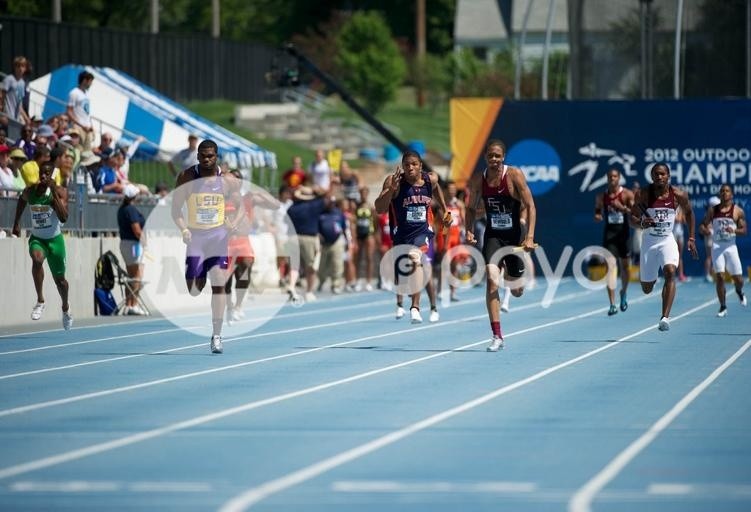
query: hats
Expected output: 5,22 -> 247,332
79,151 -> 101,167
295,186 -> 316,201
35,125 -> 55,137
0,145 -> 29,161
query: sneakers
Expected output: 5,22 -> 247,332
739,296 -> 747,307
226,278 -> 459,324
717,308 -> 728,318
608,305 -> 618,316
210,335 -> 224,354
658,317 -> 671,332
62,301 -> 73,331
487,335 -> 505,352
122,304 -> 150,316
500,301 -> 509,313
31,301 -> 46,320
619,289 -> 628,312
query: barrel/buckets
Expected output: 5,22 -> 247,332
359,149 -> 377,161
383,144 -> 401,162
408,142 -> 424,158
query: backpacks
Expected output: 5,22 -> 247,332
95,249 -> 119,292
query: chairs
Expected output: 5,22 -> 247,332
106,256 -> 152,319
